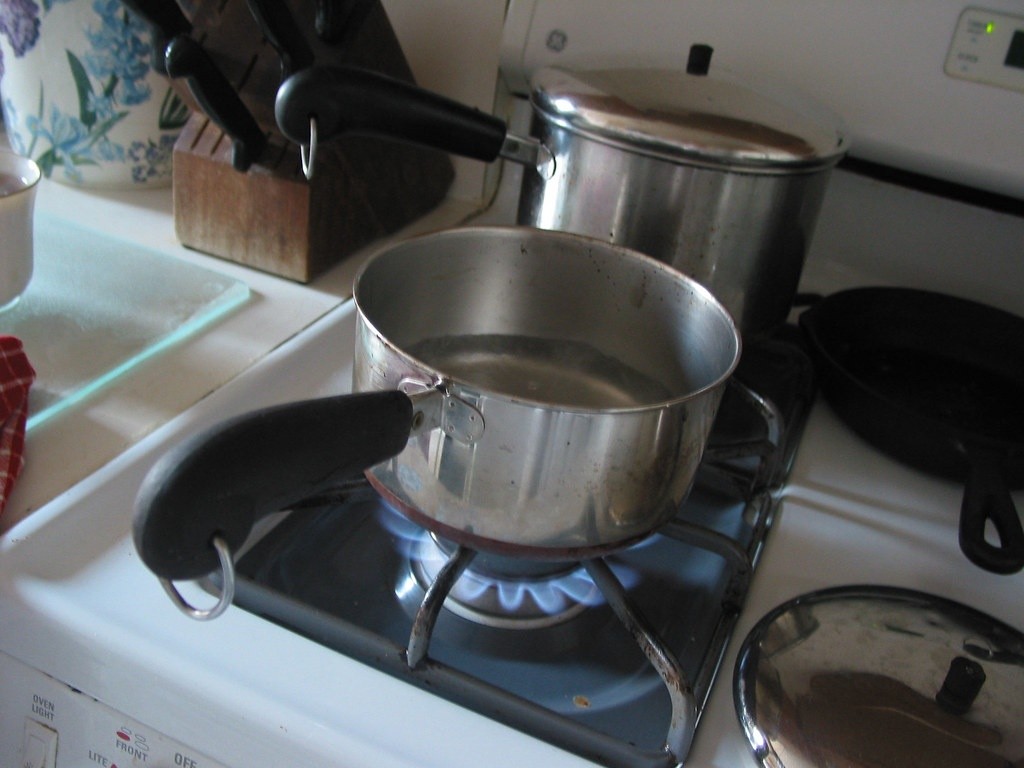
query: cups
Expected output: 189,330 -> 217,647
0,151 -> 42,306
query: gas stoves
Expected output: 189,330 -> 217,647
1,0 -> 1024,768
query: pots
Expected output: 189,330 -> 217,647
801,285 -> 1024,579
132,226 -> 743,622
271,42 -> 849,319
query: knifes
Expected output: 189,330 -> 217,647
121,0 -> 354,174
